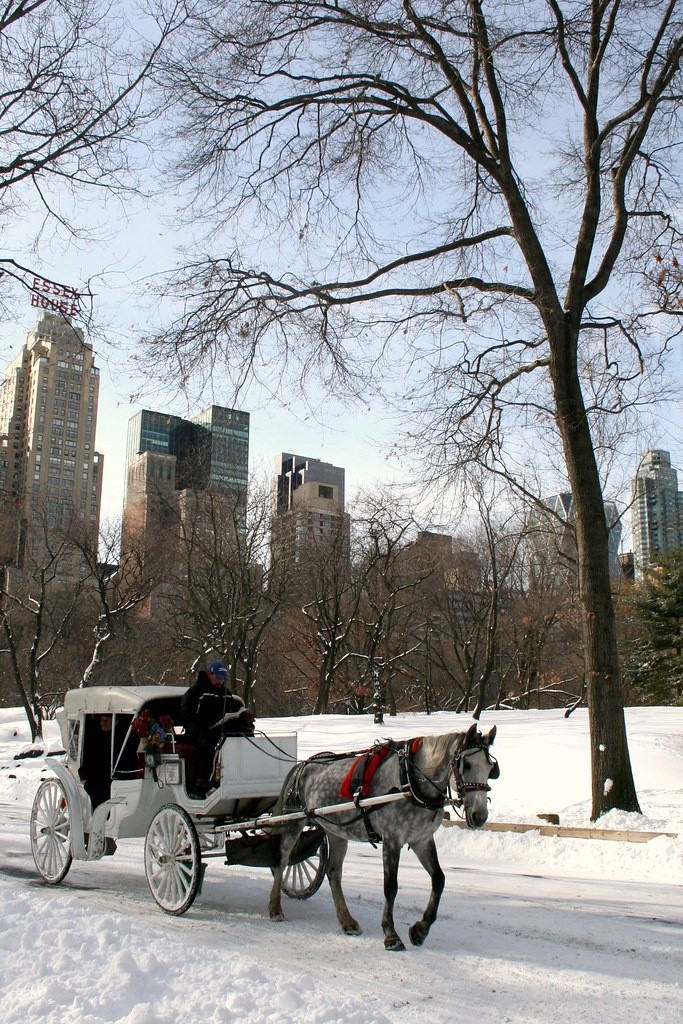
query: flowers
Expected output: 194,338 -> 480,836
131,709 -> 176,783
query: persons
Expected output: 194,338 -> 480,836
172,662 -> 253,798
82,713 -> 132,809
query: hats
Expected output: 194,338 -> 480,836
209,661 -> 228,679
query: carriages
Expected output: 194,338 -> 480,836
31,686 -> 499,952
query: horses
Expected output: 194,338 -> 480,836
269,723 -> 496,952
